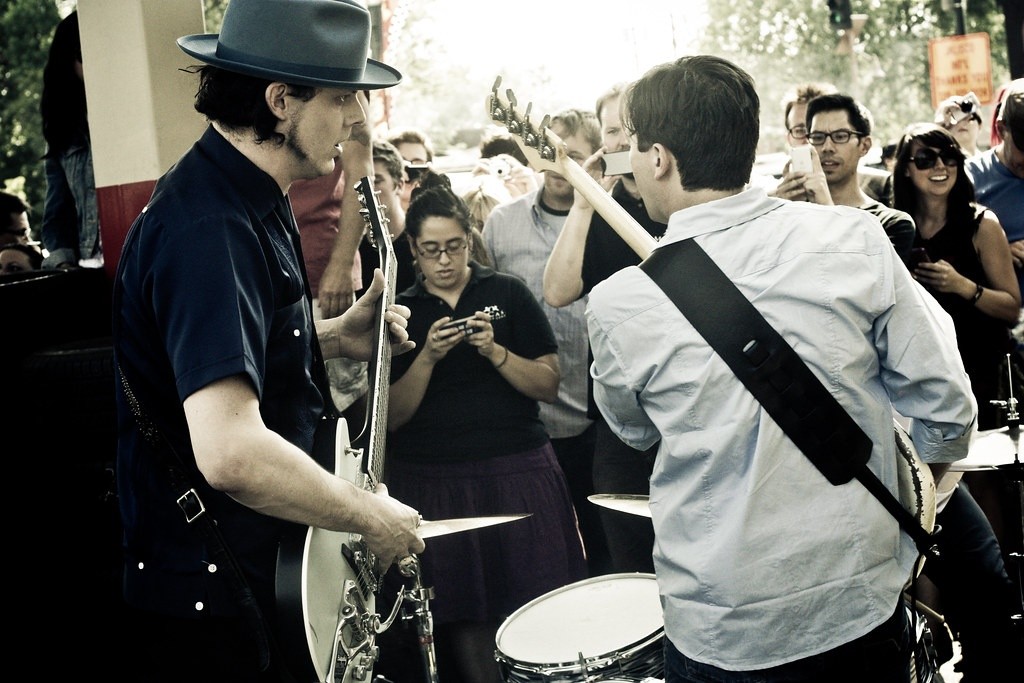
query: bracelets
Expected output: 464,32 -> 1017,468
495,347 -> 508,368
969,284 -> 983,304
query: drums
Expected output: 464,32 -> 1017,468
492,570 -> 665,683
903,598 -> 940,683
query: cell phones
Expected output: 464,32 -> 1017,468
439,315 -> 482,341
911,247 -> 931,270
601,150 -> 633,176
789,145 -> 813,174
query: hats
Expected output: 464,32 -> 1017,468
1000,78 -> 1024,152
880,144 -> 898,157
176,0 -> 402,89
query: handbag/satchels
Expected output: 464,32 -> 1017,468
997,352 -> 1024,428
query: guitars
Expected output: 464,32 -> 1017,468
483,71 -> 938,591
273,172 -> 406,683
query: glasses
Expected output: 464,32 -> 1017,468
789,127 -> 808,139
908,146 -> 960,169
806,129 -> 863,145
402,157 -> 424,165
414,235 -> 470,259
10,228 -> 30,240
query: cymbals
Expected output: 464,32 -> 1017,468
412,511 -> 537,540
586,491 -> 652,518
935,422 -> 1024,466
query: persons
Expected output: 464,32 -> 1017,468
111,1 -> 424,683
585,55 -> 978,683
0,9 -> 1024,683
